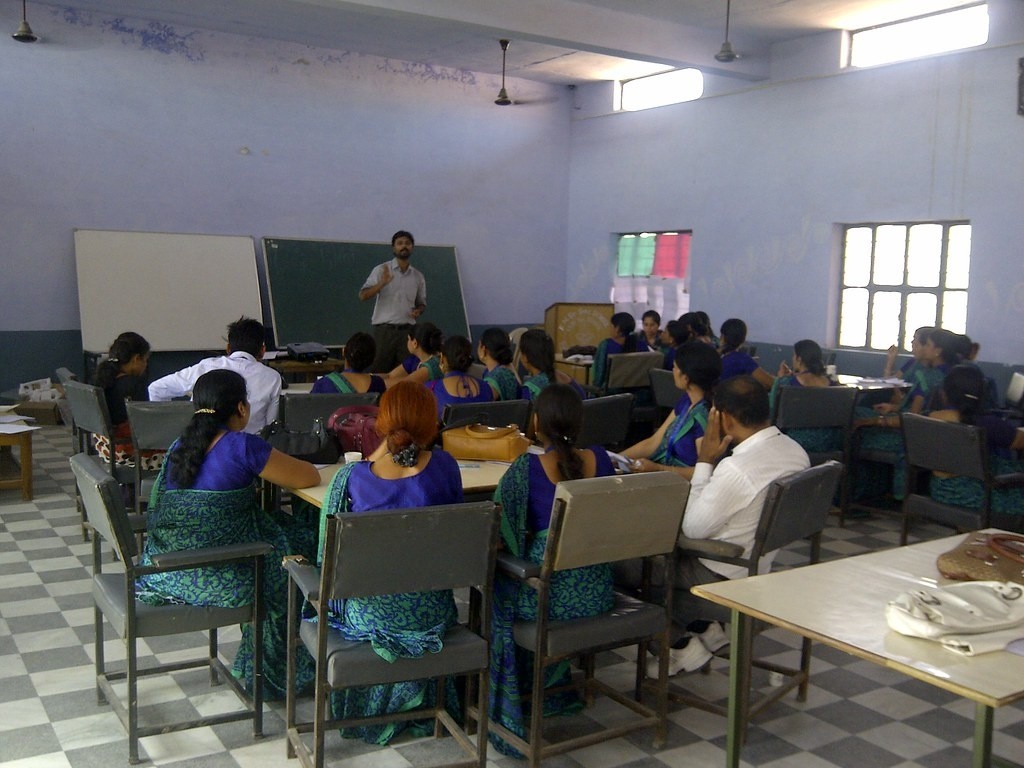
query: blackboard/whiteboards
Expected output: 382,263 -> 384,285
261,236 -> 472,350
73,226 -> 265,353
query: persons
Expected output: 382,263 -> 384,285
136,369 -> 321,700
148,319 -> 283,435
587,311 -> 775,421
928,365 -> 1024,513
770,328 -> 998,518
645,375 -> 811,679
613,341 -> 729,479
310,322 -> 586,432
302,381 -> 466,745
359,230 -> 428,374
473,384 -> 616,758
93,332 -> 166,512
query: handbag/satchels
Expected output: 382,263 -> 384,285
262,417 -> 339,464
937,530 -> 1024,584
885,581 -> 1024,656
326,405 -> 384,459
441,423 -> 530,463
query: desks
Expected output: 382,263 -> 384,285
690,525 -> 1023,768
838,373 -> 913,393
260,442 -> 546,511
0,405 -> 34,501
269,357 -> 342,375
279,382 -> 316,396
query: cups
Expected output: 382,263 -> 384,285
345,452 -> 363,465
826,364 -> 838,376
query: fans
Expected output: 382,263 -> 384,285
454,39 -> 562,106
658,0 -> 802,67
0,0 -> 103,51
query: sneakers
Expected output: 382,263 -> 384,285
646,637 -> 714,680
693,622 -> 731,655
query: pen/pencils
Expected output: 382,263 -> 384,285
458,464 -> 480,468
624,455 -> 634,466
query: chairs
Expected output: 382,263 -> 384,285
54,327 -> 1023,768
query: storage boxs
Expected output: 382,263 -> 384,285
1,387 -> 61,428
